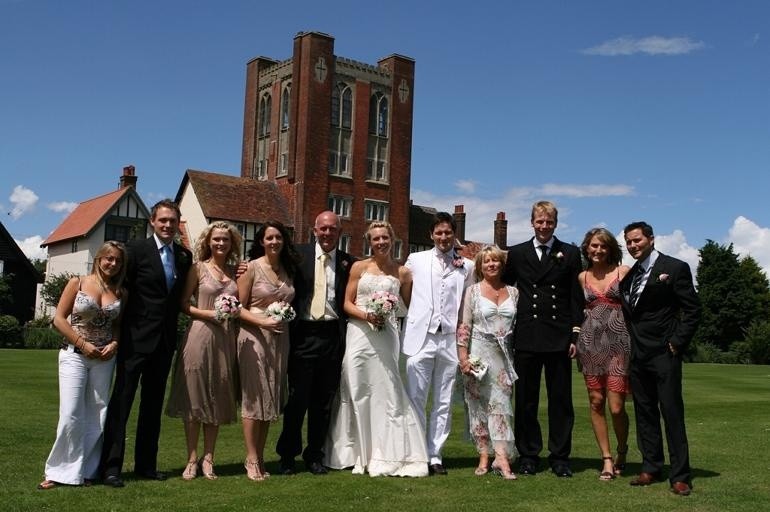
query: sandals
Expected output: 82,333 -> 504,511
182,459 -> 200,480
599,454 -> 629,482
202,459 -> 219,481
244,458 -> 270,481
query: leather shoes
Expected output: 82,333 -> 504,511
98,472 -> 126,487
304,461 -> 328,475
671,480 -> 691,495
135,468 -> 169,482
281,457 -> 296,475
628,471 -> 665,487
548,458 -> 573,478
519,455 -> 542,475
429,457 -> 449,477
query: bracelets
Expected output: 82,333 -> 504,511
364,311 -> 368,323
74,335 -> 81,346
366,313 -> 370,321
371,311 -> 373,320
457,358 -> 471,364
80,340 -> 86,352
107,341 -> 118,354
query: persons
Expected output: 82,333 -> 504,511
161,221 -> 247,481
498,200 -> 586,478
98,200 -> 194,486
575,225 -> 635,483
453,242 -> 524,481
400,209 -> 481,476
35,239 -> 130,490
234,208 -> 360,477
319,218 -> 431,478
234,217 -> 297,484
615,219 -> 706,497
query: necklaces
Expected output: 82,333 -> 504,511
209,260 -> 229,282
488,285 -> 505,300
267,267 -> 282,286
98,281 -> 110,290
372,258 -> 392,275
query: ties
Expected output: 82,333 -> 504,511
539,245 -> 551,271
308,253 -> 331,321
628,264 -> 645,306
440,255 -> 449,272
162,246 -> 174,288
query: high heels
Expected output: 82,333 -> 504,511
474,461 -> 517,481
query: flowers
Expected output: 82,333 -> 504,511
213,290 -> 242,324
263,298 -> 298,323
364,289 -> 402,334
